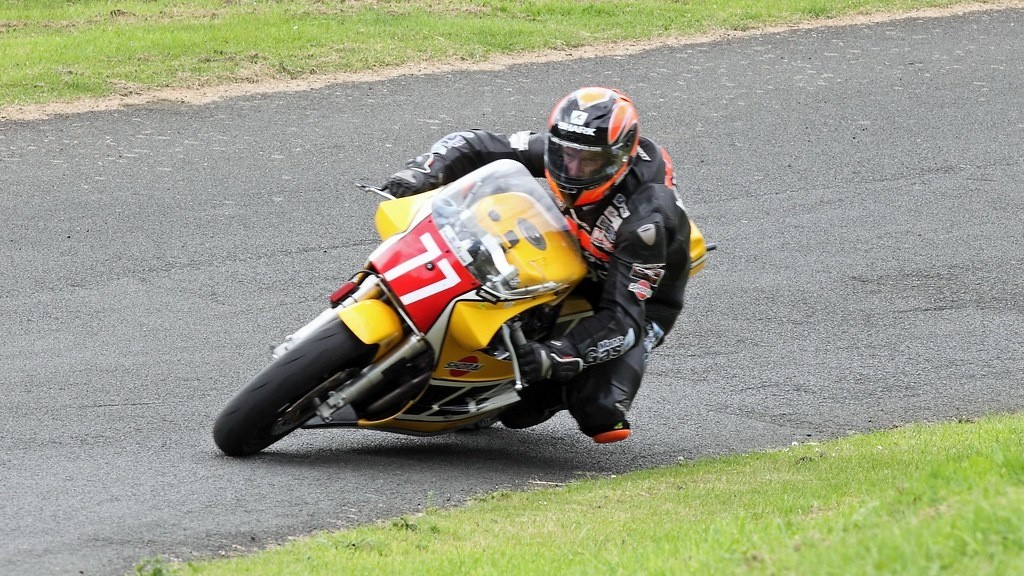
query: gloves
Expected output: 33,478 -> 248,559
517,336 -> 582,382
385,152 -> 434,195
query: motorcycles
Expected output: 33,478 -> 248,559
213,156 -> 717,457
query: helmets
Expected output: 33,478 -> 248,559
544,86 -> 638,207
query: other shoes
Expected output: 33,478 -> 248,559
502,383 -> 566,429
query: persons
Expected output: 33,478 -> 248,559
378,86 -> 692,445
